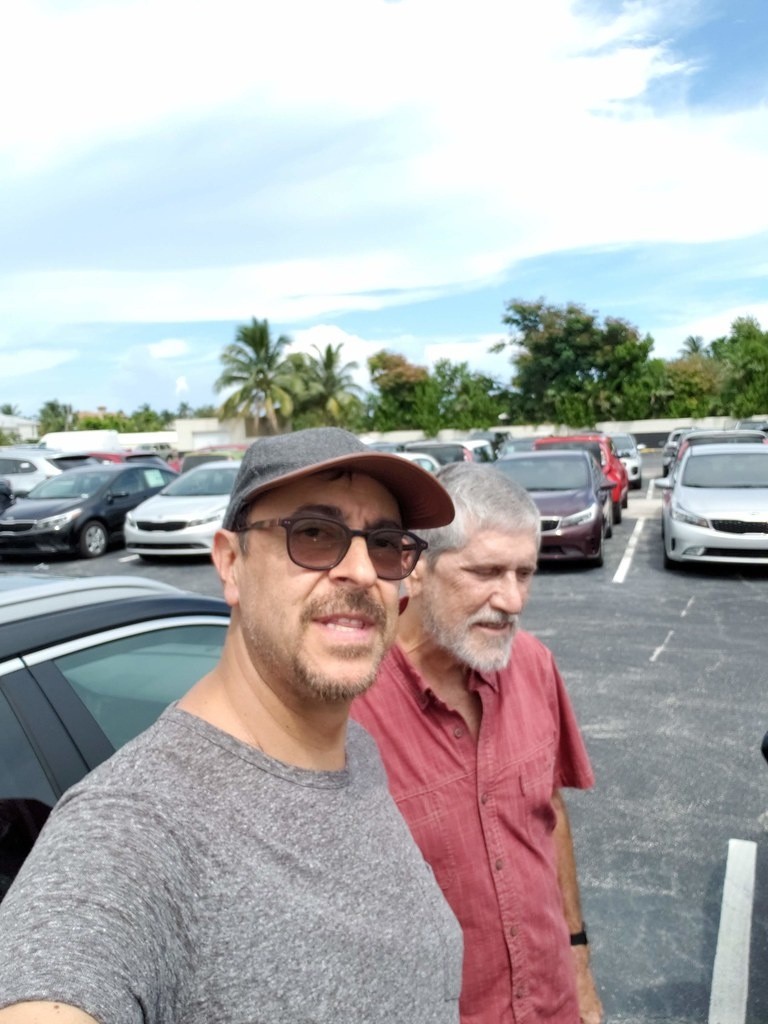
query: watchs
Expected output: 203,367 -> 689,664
569,921 -> 589,946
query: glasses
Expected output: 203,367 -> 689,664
236,513 -> 430,578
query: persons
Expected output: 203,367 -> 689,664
0,431 -> 467,1024
352,460 -> 607,1024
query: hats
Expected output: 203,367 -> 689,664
222,425 -> 457,532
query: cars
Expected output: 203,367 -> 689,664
2,398 -> 766,916
